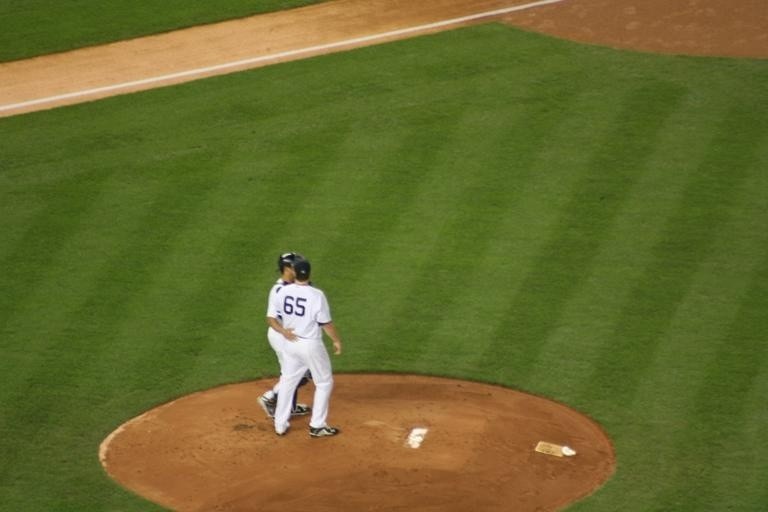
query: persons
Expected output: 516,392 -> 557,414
258,252 -> 341,437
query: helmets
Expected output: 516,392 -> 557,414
279,253 -> 310,278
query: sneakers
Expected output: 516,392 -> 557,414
308,427 -> 339,437
257,390 -> 312,435
